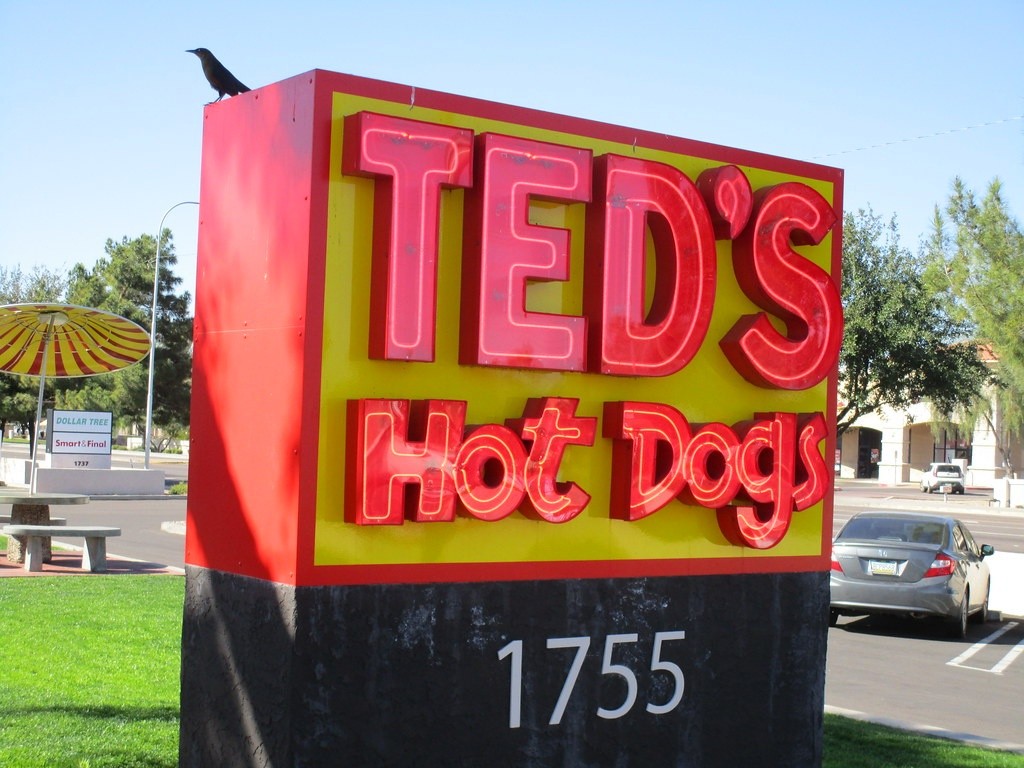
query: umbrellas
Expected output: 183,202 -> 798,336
0,301 -> 153,494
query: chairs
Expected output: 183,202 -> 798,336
913,527 -> 939,544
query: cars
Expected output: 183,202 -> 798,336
920,463 -> 966,495
830,511 -> 995,638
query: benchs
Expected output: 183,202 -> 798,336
3,525 -> 121,572
0,514 -> 67,526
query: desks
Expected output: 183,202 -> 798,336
0,491 -> 89,564
